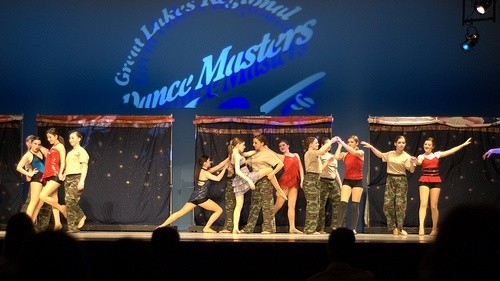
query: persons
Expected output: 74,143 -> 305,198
154,154 -> 230,233
218,131 -> 364,235
16,128 -> 90,233
360,134 -> 472,237
2,211 -> 372,281
482,147 -> 500,160
